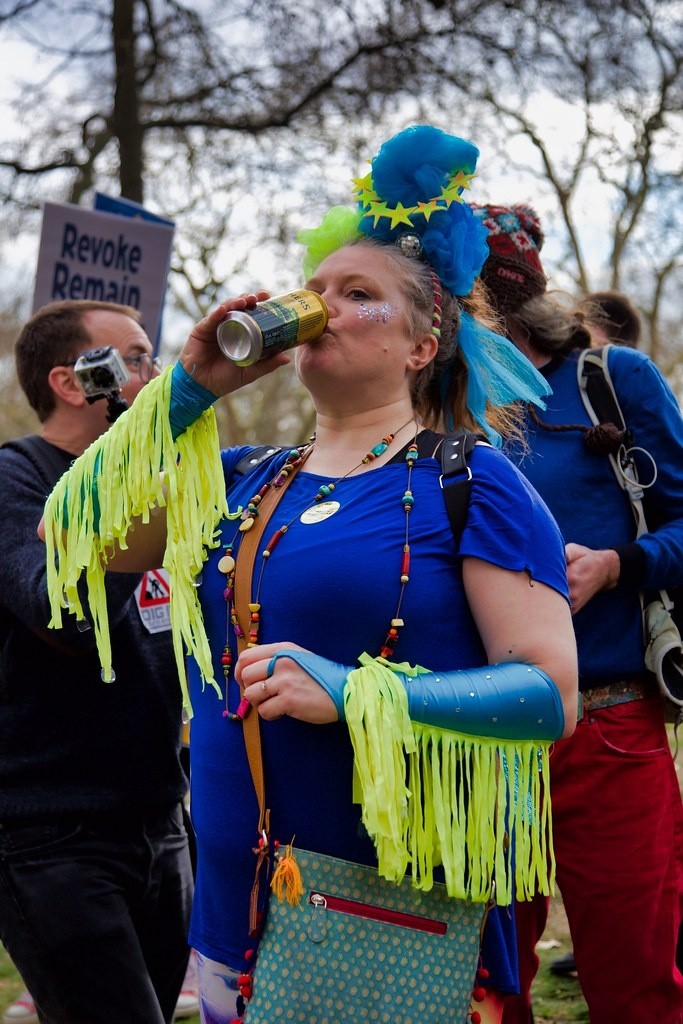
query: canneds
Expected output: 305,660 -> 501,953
217,289 -> 330,366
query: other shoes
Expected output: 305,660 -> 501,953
3,989 -> 37,1023
550,952 -> 578,977
175,985 -> 200,1019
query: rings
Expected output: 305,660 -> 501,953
262,681 -> 271,699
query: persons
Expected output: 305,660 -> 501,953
39,234 -> 579,1024
483,204 -> 682,1024
1,299 -> 193,1023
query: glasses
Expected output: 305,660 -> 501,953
62,352 -> 162,384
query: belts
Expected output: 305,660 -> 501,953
577,682 -> 647,721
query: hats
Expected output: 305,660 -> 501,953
468,201 -> 548,309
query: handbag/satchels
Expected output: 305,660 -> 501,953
243,844 -> 495,1024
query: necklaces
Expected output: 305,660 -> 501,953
218,423 -> 418,668
223,419 -> 415,720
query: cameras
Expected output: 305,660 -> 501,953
72,346 -> 131,426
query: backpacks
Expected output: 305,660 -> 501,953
577,343 -> 683,722
